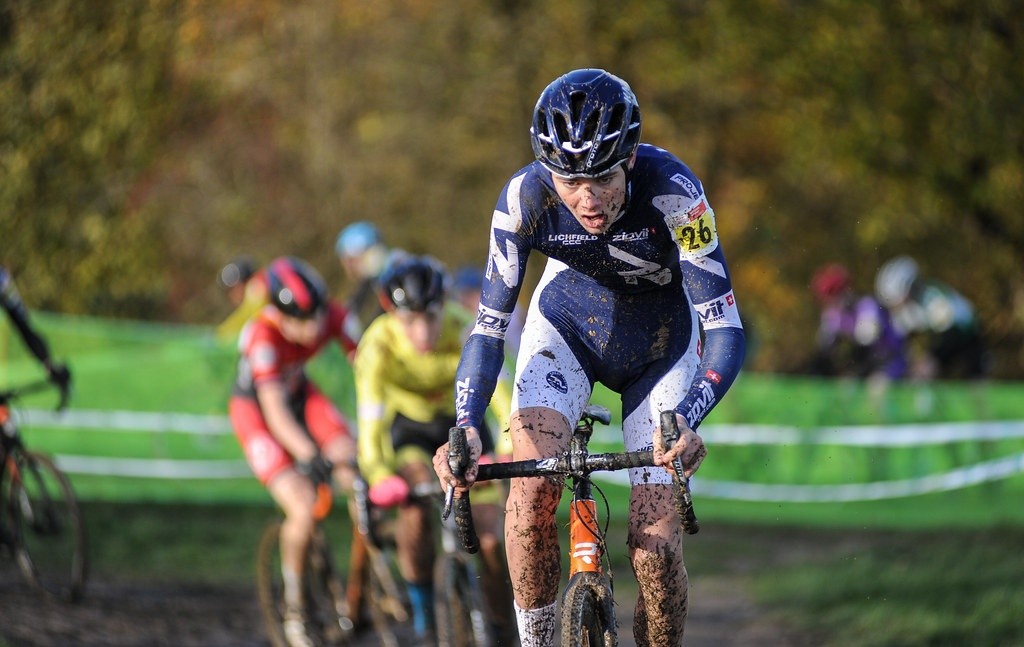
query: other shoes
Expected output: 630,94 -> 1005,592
284,606 -> 321,647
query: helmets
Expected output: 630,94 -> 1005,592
262,254 -> 327,319
530,67 -> 641,177
876,256 -> 918,305
381,254 -> 445,314
814,264 -> 848,297
456,266 -> 480,290
338,221 -> 378,255
221,261 -> 251,291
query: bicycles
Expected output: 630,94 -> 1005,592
253,455 -> 440,647
440,405 -> 699,647
352,475 -> 512,647
0,367 -> 93,606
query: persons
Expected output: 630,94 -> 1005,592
432,68 -> 746,647
228,220 -> 526,647
0,265 -> 68,486
812,255 -> 994,419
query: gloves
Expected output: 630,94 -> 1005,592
370,475 -> 412,508
